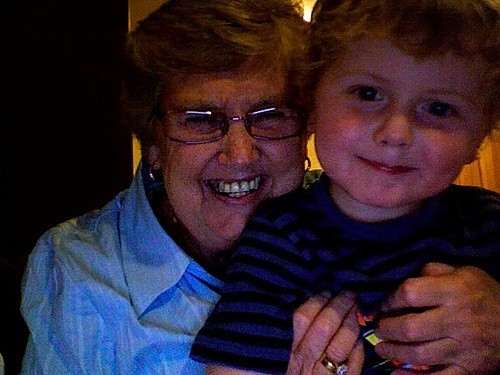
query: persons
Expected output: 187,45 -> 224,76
190,0 -> 500,375
19,0 -> 500,375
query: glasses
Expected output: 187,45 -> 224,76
148,102 -> 307,146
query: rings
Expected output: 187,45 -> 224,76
320,353 -> 341,374
